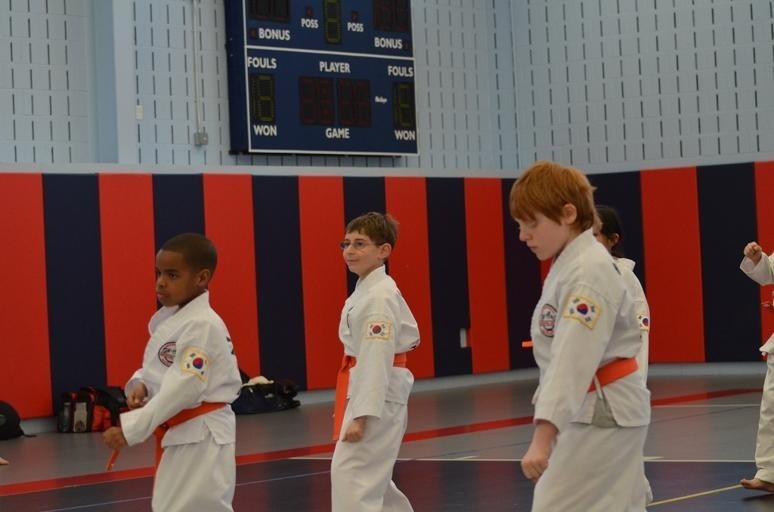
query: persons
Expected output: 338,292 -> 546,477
329,210 -> 421,512
101,231 -> 244,511
591,202 -> 654,504
738,241 -> 774,494
508,160 -> 647,512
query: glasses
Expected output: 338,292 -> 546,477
339,241 -> 374,251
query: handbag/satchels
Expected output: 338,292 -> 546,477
233,370 -> 301,414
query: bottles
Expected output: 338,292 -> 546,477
459,329 -> 470,375
62,401 -> 72,432
